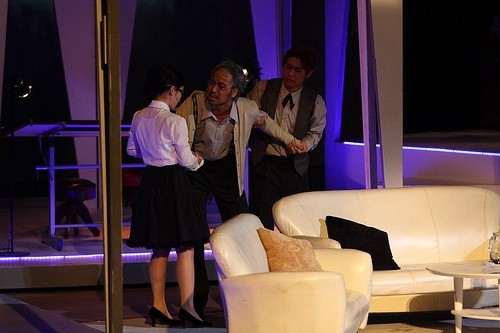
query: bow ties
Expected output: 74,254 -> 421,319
282,93 -> 295,110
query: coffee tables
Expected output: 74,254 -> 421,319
424,258 -> 500,333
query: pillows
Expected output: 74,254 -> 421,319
258,227 -> 324,273
325,214 -> 401,270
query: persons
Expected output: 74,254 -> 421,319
248,45 -> 326,232
127,71 -> 212,329
175,61 -> 306,319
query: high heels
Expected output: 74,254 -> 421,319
150,306 -> 182,328
178,307 -> 208,329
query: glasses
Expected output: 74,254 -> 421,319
207,79 -> 236,91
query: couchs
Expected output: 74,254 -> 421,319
208,213 -> 372,333
271,186 -> 500,313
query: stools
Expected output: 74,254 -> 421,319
48,176 -> 101,240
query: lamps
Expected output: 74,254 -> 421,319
0,73 -> 32,257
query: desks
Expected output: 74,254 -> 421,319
5,119 -> 132,252
